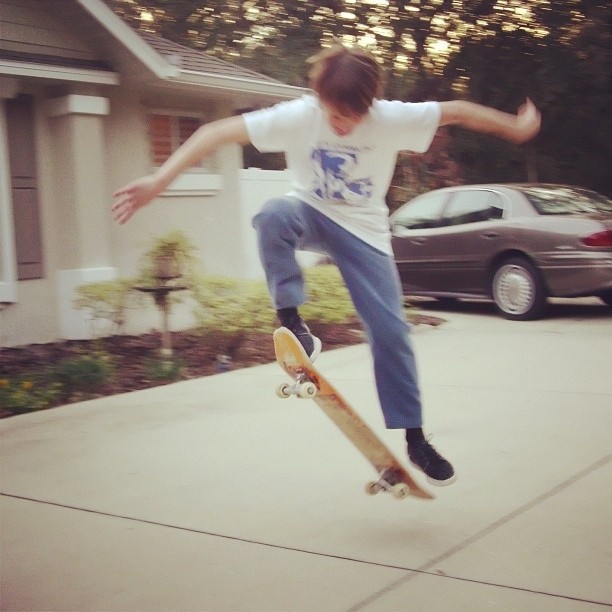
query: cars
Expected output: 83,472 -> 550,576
313,181 -> 612,322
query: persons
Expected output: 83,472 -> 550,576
111,47 -> 541,486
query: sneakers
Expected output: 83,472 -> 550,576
286,316 -> 323,363
407,432 -> 457,485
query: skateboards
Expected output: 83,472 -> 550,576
273,327 -> 436,500
